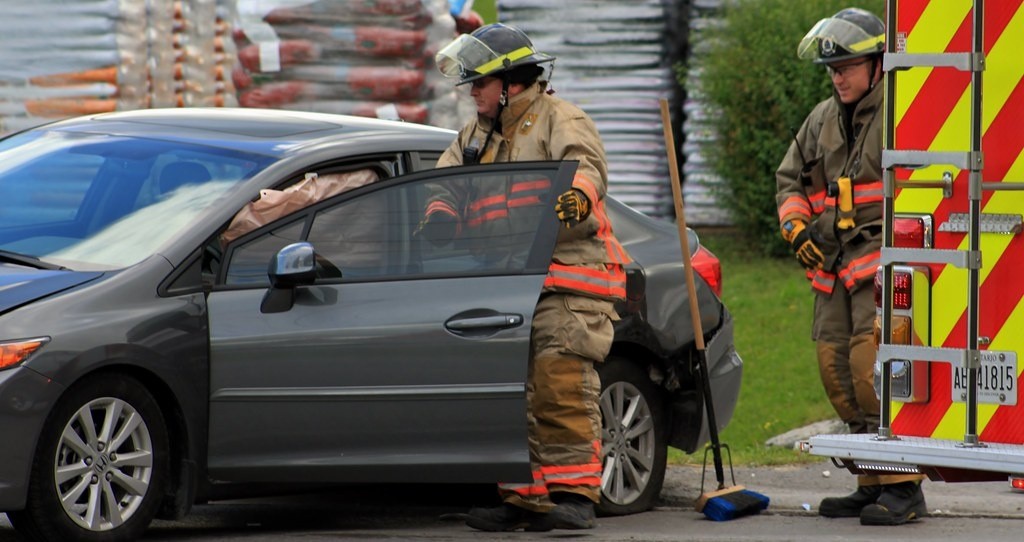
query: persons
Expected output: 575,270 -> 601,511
412,22 -> 635,533
775,8 -> 927,526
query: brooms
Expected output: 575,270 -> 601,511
651,95 -> 769,522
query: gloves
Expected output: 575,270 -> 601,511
782,219 -> 825,274
413,211 -> 459,248
555,188 -> 590,229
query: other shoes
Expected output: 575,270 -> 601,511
818,484 -> 882,517
548,493 -> 598,529
860,480 -> 927,525
465,502 -> 555,532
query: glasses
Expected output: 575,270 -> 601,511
825,59 -> 871,78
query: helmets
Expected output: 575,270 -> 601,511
797,7 -> 885,63
435,23 -> 557,86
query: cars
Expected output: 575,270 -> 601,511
0,105 -> 745,541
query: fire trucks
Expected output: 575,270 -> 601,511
759,1 -> 1024,496
